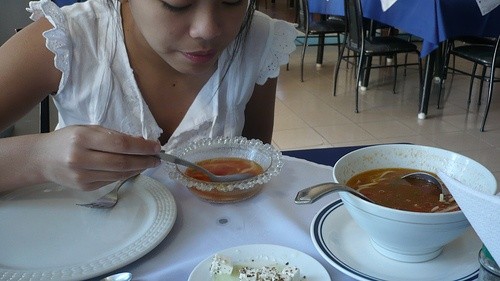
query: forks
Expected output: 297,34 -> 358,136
73,149 -> 166,210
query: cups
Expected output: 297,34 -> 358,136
477,242 -> 500,281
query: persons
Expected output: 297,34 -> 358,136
0,0 -> 301,193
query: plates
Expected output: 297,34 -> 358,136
187,244 -> 331,281
310,197 -> 484,281
0,172 -> 178,281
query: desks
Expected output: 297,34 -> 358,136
308,0 -> 500,119
91,142 -> 412,281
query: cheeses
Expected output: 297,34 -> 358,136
210,254 -> 300,281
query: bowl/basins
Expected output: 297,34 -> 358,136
164,135 -> 284,204
332,144 -> 498,264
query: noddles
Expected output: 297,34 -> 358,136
346,168 -> 462,212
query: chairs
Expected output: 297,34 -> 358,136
286,0 -> 500,132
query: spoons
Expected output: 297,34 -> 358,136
100,272 -> 133,281
154,153 -> 255,182
